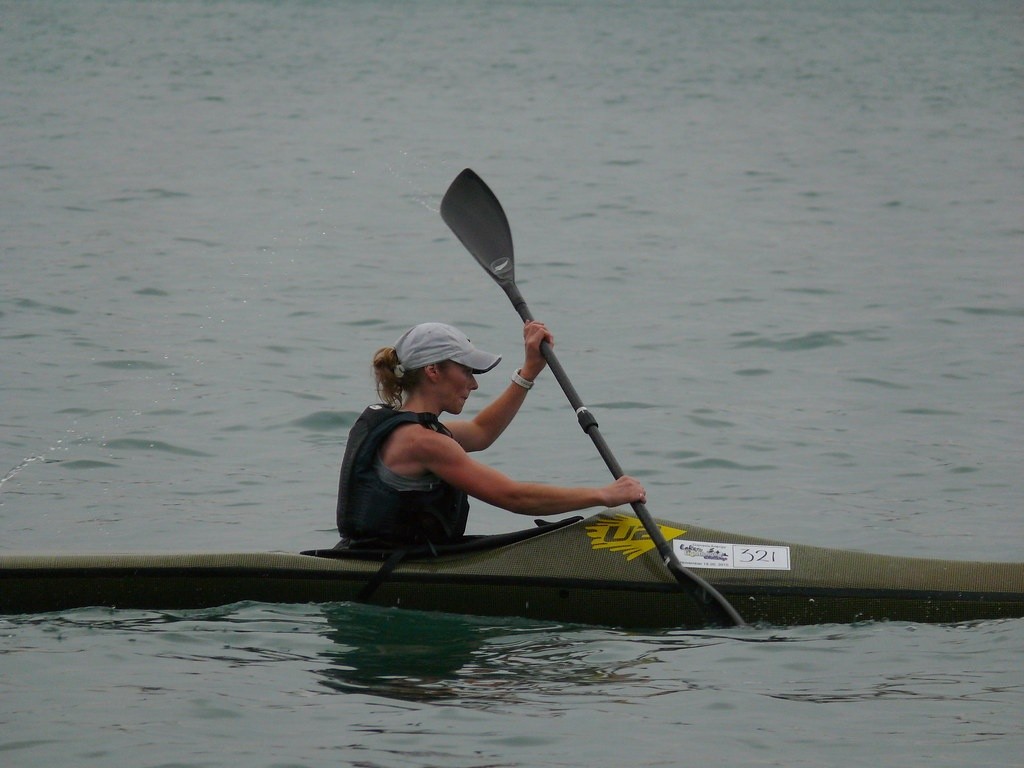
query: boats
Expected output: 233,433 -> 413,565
0,502 -> 1024,626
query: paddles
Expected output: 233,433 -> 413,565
438,165 -> 749,628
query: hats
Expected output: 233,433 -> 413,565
393,322 -> 503,374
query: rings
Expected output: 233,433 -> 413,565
639,492 -> 642,496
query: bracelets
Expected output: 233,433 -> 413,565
512,368 -> 534,390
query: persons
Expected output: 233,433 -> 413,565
335,320 -> 647,551
